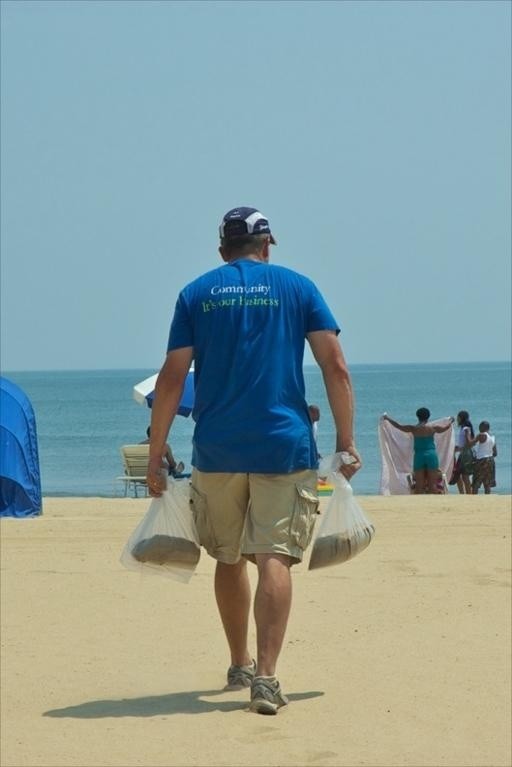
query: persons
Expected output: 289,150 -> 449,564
145,204 -> 364,716
139,425 -> 185,473
465,420 -> 498,494
454,410 -> 477,494
308,404 -> 324,460
383,407 -> 455,494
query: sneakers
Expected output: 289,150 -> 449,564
227,658 -> 290,716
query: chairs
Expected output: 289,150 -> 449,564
117,443 -> 151,497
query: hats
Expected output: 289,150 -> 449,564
219,207 -> 278,246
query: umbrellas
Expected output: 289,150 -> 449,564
131,368 -> 195,420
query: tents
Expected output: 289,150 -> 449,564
2,376 -> 45,525
